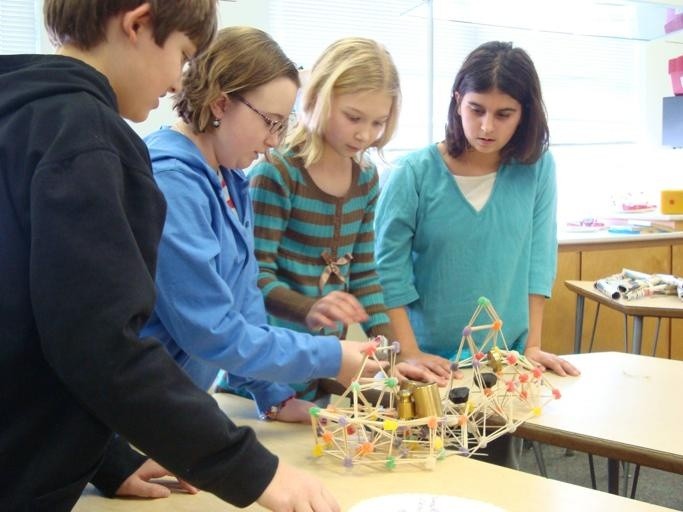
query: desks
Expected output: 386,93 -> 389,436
71,392 -> 669,508
321,351 -> 683,499
565,279 -> 683,460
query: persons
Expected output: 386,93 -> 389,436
373,40 -> 582,381
0,0 -> 343,512
247,37 -> 448,388
142,25 -> 389,424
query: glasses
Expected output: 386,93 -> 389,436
229,91 -> 288,137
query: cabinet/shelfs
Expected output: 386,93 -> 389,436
671,246 -> 683,360
539,253 -> 580,354
580,243 -> 671,357
